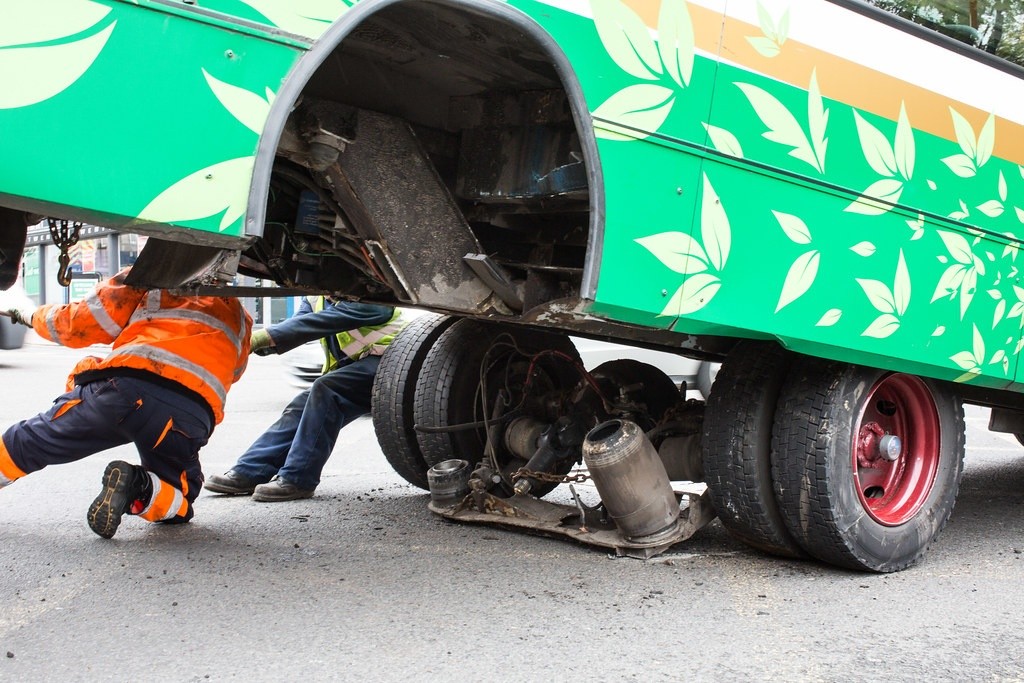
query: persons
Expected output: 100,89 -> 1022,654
0,265 -> 254,539
204,295 -> 412,502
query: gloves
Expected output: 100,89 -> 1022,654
9,306 -> 34,328
248,329 -> 269,354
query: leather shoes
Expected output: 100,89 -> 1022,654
206,471 -> 254,497
255,478 -> 315,502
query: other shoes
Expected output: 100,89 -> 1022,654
86,460 -> 148,540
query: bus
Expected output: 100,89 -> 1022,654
0,0 -> 1024,584
